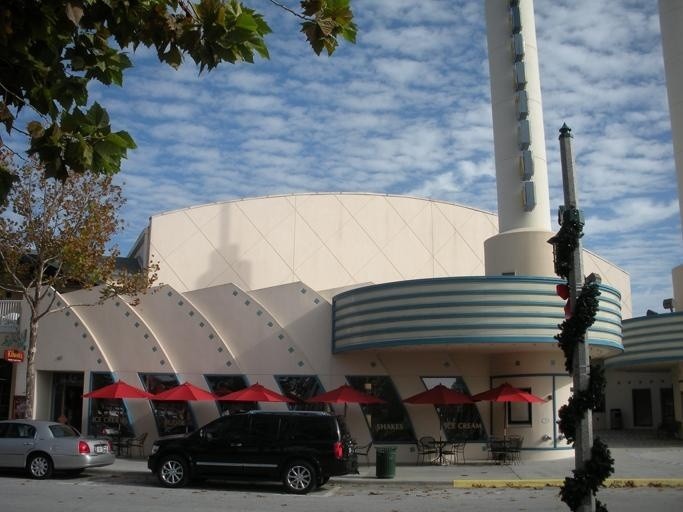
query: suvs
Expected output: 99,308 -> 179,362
146,407 -> 341,494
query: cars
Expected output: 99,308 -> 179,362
0,418 -> 116,479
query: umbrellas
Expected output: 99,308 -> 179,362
146,381 -> 221,434
302,383 -> 385,418
399,382 -> 480,441
471,381 -> 548,448
215,381 -> 298,410
81,378 -> 155,456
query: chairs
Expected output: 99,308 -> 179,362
416,432 -> 467,465
485,432 -> 524,466
353,440 -> 372,470
94,431 -> 147,461
